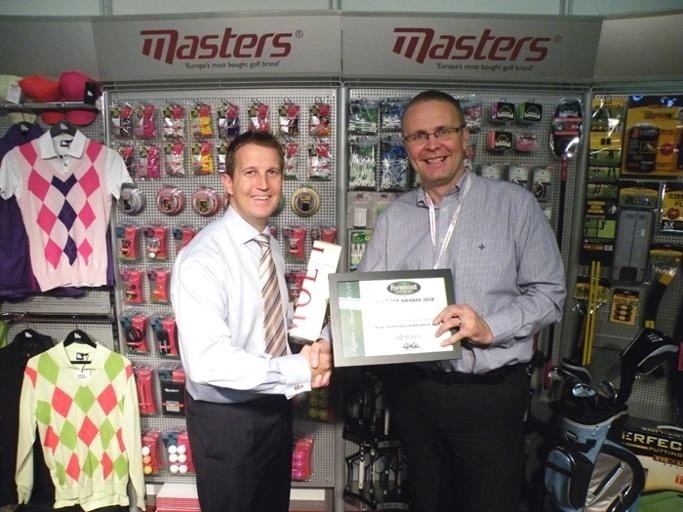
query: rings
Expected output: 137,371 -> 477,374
457,318 -> 464,326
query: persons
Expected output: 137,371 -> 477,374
170,132 -> 333,511
309,90 -> 567,510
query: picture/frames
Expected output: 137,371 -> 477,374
328,268 -> 462,369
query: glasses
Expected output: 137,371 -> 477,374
405,124 -> 466,142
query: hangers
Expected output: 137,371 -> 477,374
63,313 -> 97,364
20,309 -> 40,344
16,101 -> 34,132
50,102 -> 77,137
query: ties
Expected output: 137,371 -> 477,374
252,234 -> 287,359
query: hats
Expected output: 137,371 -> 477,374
59,72 -> 101,125
18,74 -> 64,125
0,74 -> 38,124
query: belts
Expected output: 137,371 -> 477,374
411,362 -> 527,385
